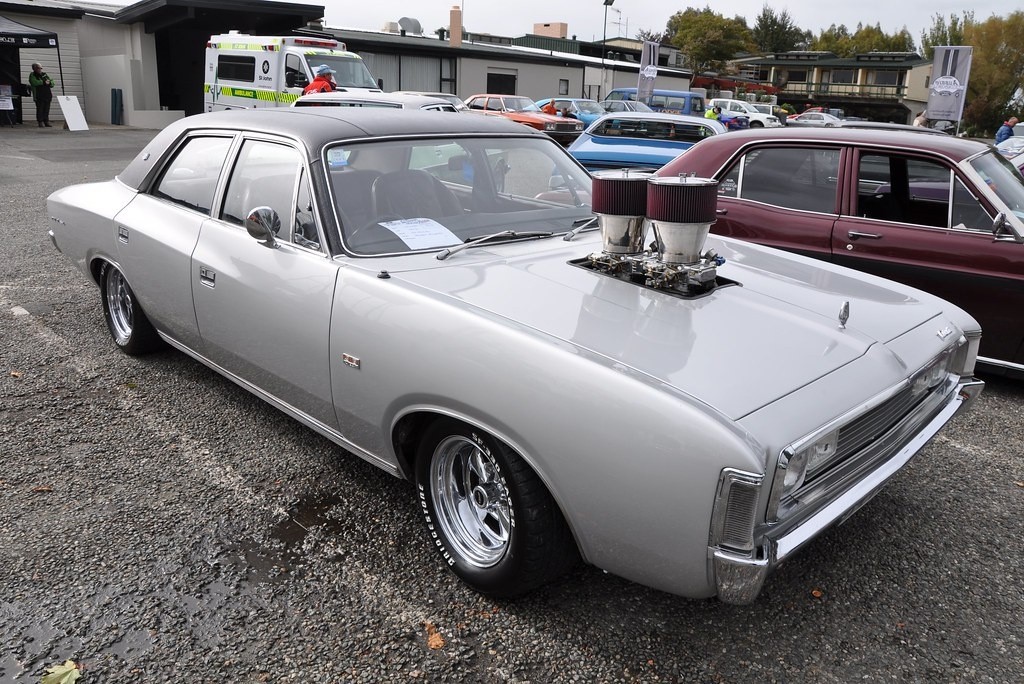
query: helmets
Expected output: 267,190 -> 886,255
32,63 -> 42,70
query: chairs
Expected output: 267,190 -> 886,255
372,167 -> 464,228
332,167 -> 386,230
242,174 -> 297,241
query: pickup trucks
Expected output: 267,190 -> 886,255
786,107 -> 822,119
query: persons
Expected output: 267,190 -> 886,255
29,63 -> 56,127
302,64 -> 340,106
912,109 -> 928,127
543,99 -> 557,115
701,106 -> 721,135
561,107 -> 577,119
995,117 -> 1018,145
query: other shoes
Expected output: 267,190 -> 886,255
38,122 -> 43,127
44,122 -> 52,127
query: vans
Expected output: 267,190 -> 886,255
604,89 -> 705,118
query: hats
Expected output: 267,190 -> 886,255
715,106 -> 721,114
317,65 -> 336,75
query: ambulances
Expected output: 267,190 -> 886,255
203,29 -> 384,114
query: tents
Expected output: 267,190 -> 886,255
0,14 -> 65,96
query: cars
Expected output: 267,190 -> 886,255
289,93 -> 460,113
654,129 -> 1024,372
42,113 -> 982,613
524,99 -> 622,136
559,111 -> 727,170
785,111 -> 842,128
598,99 -> 654,112
709,99 -> 783,128
456,93 -> 585,146
393,90 -> 498,116
